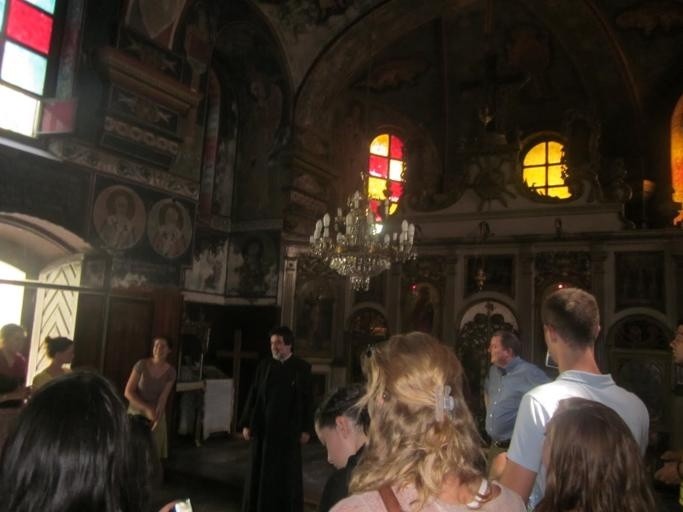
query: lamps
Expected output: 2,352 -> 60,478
308,188 -> 418,292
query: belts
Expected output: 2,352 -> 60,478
494,440 -> 509,448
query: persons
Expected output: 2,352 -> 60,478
405,287 -> 432,332
491,287 -> 651,512
314,382 -> 372,512
653,319 -> 682,512
123,336 -> 176,488
0,370 -> 191,512
150,203 -> 189,254
239,326 -> 313,512
531,396 -> 683,512
327,331 -> 530,512
99,188 -> 141,249
0,324 -> 29,414
482,330 -> 552,456
32,336 -> 76,389
